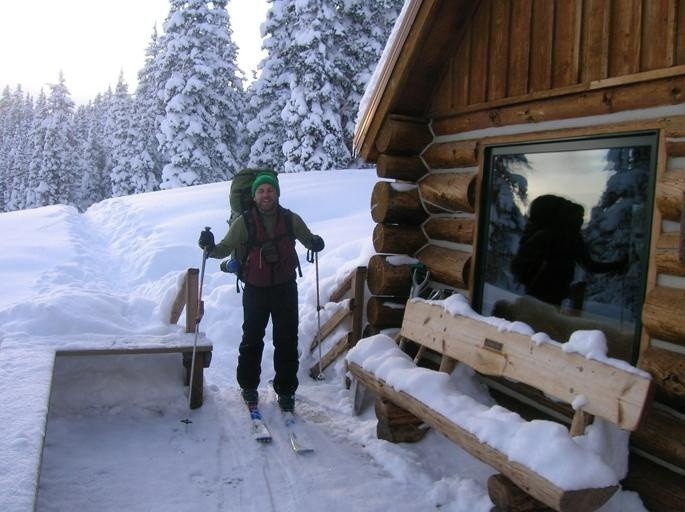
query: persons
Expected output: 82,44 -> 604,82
526,218 -> 614,306
198,170 -> 326,410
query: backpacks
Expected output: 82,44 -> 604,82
227,167 -> 294,283
512,193 -> 577,287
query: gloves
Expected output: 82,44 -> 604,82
309,235 -> 325,252
614,257 -> 633,276
198,231 -> 214,253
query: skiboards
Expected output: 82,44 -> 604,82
248,379 -> 314,454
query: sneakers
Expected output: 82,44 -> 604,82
277,392 -> 295,412
241,388 -> 259,404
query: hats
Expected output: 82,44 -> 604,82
572,203 -> 584,220
252,171 -> 281,198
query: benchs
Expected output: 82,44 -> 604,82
346,297 -> 655,512
477,298 -> 636,364
